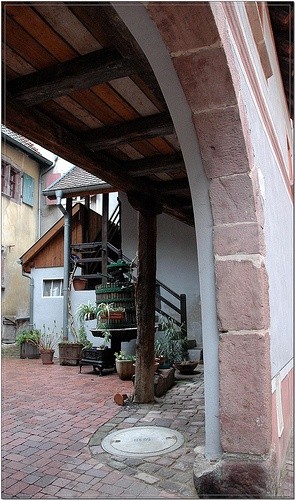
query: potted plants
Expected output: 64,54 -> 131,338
75,301 -> 97,320
58,339 -> 84,365
90,323 -> 112,341
72,277 -> 87,290
14,328 -> 41,359
40,320 -> 61,364
97,302 -> 126,321
176,359 -> 199,373
78,341 -> 117,375
161,316 -> 190,364
112,349 -> 134,380
154,350 -> 172,368
